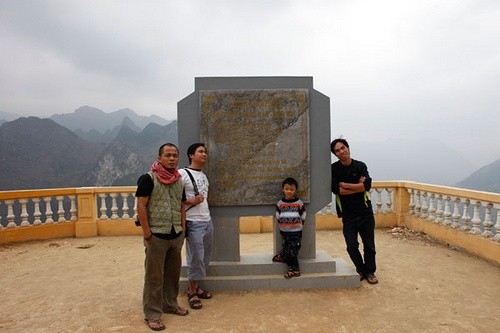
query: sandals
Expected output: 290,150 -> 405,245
272,250 -> 286,262
284,269 -> 301,278
186,286 -> 213,309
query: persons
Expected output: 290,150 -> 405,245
330,139 -> 379,285
177,142 -> 215,308
135,143 -> 189,331
272,177 -> 306,279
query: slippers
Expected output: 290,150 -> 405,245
357,271 -> 378,284
143,318 -> 166,331
163,305 -> 189,316
